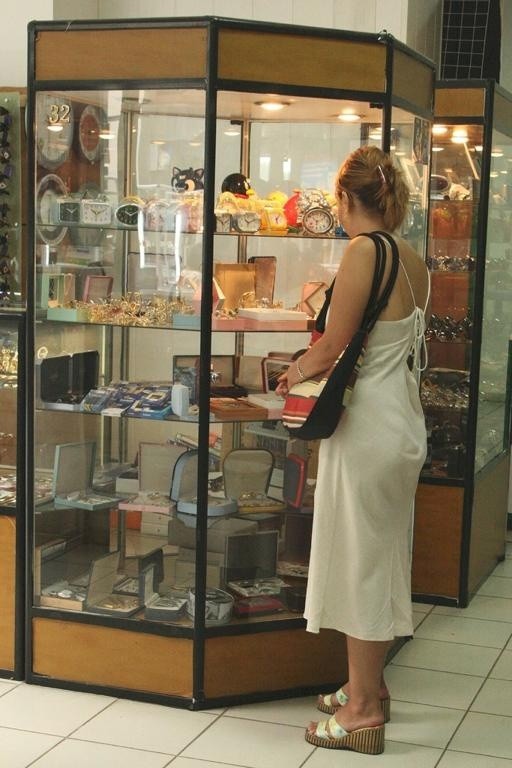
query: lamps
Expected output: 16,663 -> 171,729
45,116 -> 65,132
450,129 -> 469,145
86,118 -> 115,140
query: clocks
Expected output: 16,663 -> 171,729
55,198 -> 82,225
232,211 -> 259,233
260,205 -> 289,237
214,211 -> 232,234
297,188 -> 335,238
115,203 -> 143,228
81,199 -> 113,226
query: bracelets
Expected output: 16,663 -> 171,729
296,354 -> 311,381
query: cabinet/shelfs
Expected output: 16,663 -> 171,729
23,17 -> 437,712
403,78 -> 512,608
0,86 -> 29,682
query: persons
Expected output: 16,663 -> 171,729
271,142 -> 435,757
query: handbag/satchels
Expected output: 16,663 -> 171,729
280,325 -> 369,440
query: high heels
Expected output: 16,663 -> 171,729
305,714 -> 385,756
316,684 -> 392,724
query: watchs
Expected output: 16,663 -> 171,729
421,250 -> 476,472
